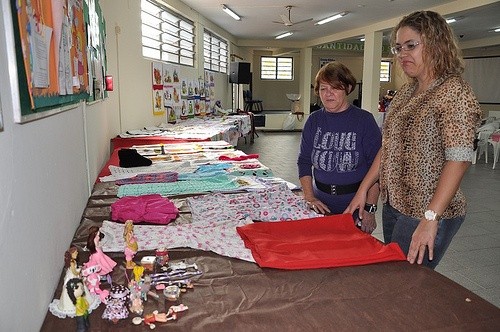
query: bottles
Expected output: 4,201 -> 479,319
156,243 -> 170,266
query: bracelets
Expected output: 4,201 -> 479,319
364,203 -> 377,212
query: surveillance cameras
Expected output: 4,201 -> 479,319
460,34 -> 464,38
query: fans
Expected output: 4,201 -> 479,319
271,6 -> 314,27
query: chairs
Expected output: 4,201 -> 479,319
469,126 -> 495,164
487,130 -> 500,169
242,90 -> 263,114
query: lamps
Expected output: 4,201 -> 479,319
274,32 -> 293,40
220,4 -> 241,21
314,11 -> 347,26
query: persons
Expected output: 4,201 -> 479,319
124,221 -> 138,267
297,62 -> 382,234
67,279 -> 89,332
102,285 -> 129,322
49,247 -> 102,318
132,308 -> 177,329
82,227 -> 117,275
343,11 -> 483,269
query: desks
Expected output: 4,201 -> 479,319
40,113 -> 500,332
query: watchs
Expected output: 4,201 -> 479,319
424,210 -> 441,221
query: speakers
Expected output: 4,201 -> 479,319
229,62 -> 251,84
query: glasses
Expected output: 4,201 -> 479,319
391,39 -> 425,55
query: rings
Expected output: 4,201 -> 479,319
312,204 -> 315,207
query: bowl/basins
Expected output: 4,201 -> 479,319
285,94 -> 301,101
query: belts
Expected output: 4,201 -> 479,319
313,178 -> 363,196
